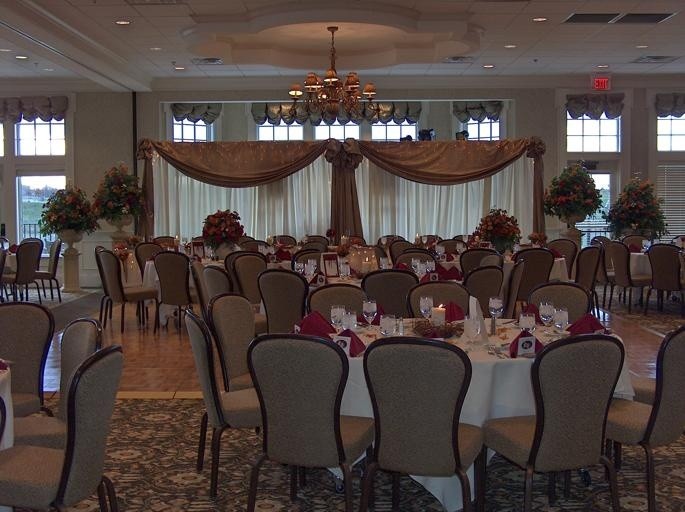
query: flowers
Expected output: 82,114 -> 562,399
469,203 -> 524,250
91,159 -> 153,224
35,184 -> 102,237
541,159 -> 602,221
600,176 -> 671,237
197,208 -> 247,246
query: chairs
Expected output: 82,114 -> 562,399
0,231 -> 685,511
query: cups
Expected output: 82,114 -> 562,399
380,237 -> 387,245
328,292 -> 569,342
293,256 -> 437,281
267,235 -> 273,245
434,239 -> 519,261
167,244 -> 278,263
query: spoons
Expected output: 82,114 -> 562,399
488,346 -> 509,359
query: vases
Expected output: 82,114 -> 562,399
56,228 -> 83,251
623,227 -> 653,236
557,214 -> 588,230
104,216 -> 135,239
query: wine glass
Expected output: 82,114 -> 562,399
422,237 -> 427,249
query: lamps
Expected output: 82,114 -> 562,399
285,22 -> 380,118
